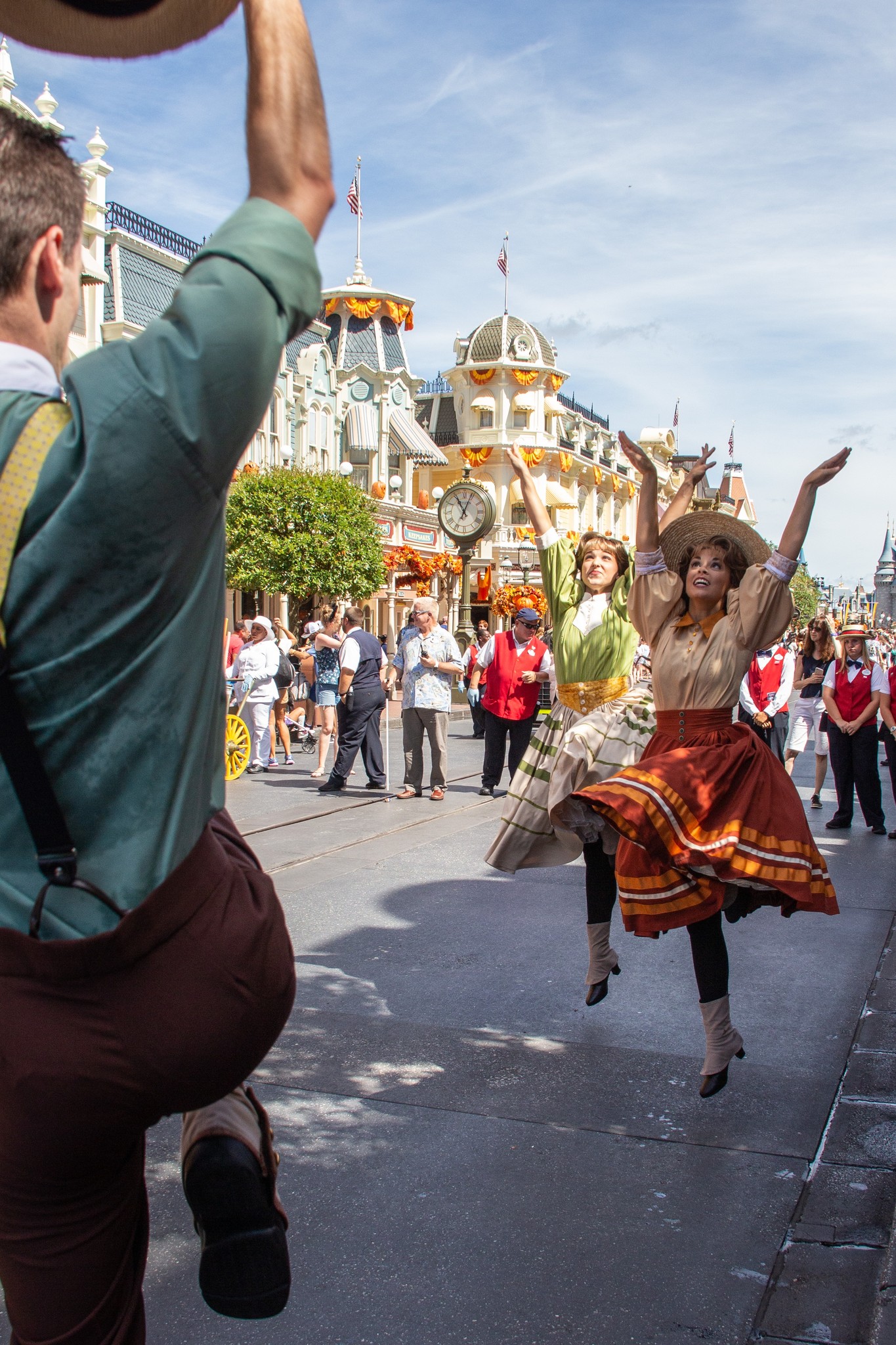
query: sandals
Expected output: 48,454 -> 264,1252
310,767 -> 355,778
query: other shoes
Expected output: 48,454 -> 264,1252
881,759 -> 889,766
887,828 -> 896,840
181,1088 -> 292,1319
479,785 -> 513,796
298,728 -> 315,739
472,733 -> 485,739
826,819 -> 887,834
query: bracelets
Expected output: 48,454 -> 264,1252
338,692 -> 347,696
292,650 -> 296,655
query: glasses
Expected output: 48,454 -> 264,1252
518,619 -> 539,629
809,626 -> 823,632
411,610 -> 428,616
478,625 -> 484,627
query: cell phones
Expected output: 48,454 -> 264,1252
815,667 -> 824,675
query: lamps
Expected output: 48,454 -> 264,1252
389,475 -> 403,496
432,486 -> 444,506
278,445 -> 294,470
339,461 -> 353,480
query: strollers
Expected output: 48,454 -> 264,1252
276,716 -> 318,754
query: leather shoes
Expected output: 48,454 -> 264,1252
396,790 -> 445,800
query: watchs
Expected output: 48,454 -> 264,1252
890,726 -> 896,734
433,661 -> 439,670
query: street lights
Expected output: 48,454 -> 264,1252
854,606 -> 871,624
810,577 -> 825,617
500,554 -> 513,631
880,610 -> 891,633
513,535 -> 539,588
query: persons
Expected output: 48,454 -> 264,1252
628,639 -> 652,691
738,634 -> 795,768
778,625 -> 896,765
784,615 -> 842,808
225,602 -> 417,792
821,625 -> 887,835
382,596 -> 465,800
879,665 -> 896,840
0,0 -> 333,1345
484,443 -> 717,1005
457,619 -> 559,739
440,616 -> 448,630
550,431 -> 853,1098
467,607 -> 551,795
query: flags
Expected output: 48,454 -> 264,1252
728,426 -> 734,457
497,241 -> 509,278
673,401 -> 679,427
347,169 -> 363,220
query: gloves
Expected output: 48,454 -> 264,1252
458,681 -> 465,693
466,688 -> 479,708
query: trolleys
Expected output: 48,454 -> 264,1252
223,678 -> 256,780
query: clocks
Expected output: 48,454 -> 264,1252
440,489 -> 484,536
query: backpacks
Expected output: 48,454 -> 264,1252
272,638 -> 311,702
783,642 -> 792,651
540,631 -> 553,653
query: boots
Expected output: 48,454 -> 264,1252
698,994 -> 746,1098
584,920 -> 621,1006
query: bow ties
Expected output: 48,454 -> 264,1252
847,660 -> 862,670
757,650 -> 772,658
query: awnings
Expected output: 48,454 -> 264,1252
388,409 -> 449,466
345,405 -> 378,453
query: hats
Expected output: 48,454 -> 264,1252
658,509 -> 773,577
515,607 -> 543,621
244,616 -> 275,640
0,0 -> 246,60
301,621 -> 324,638
835,624 -> 872,642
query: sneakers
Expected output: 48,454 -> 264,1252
319,781 -> 386,792
268,755 -> 294,766
810,795 -> 823,808
236,764 -> 269,774
